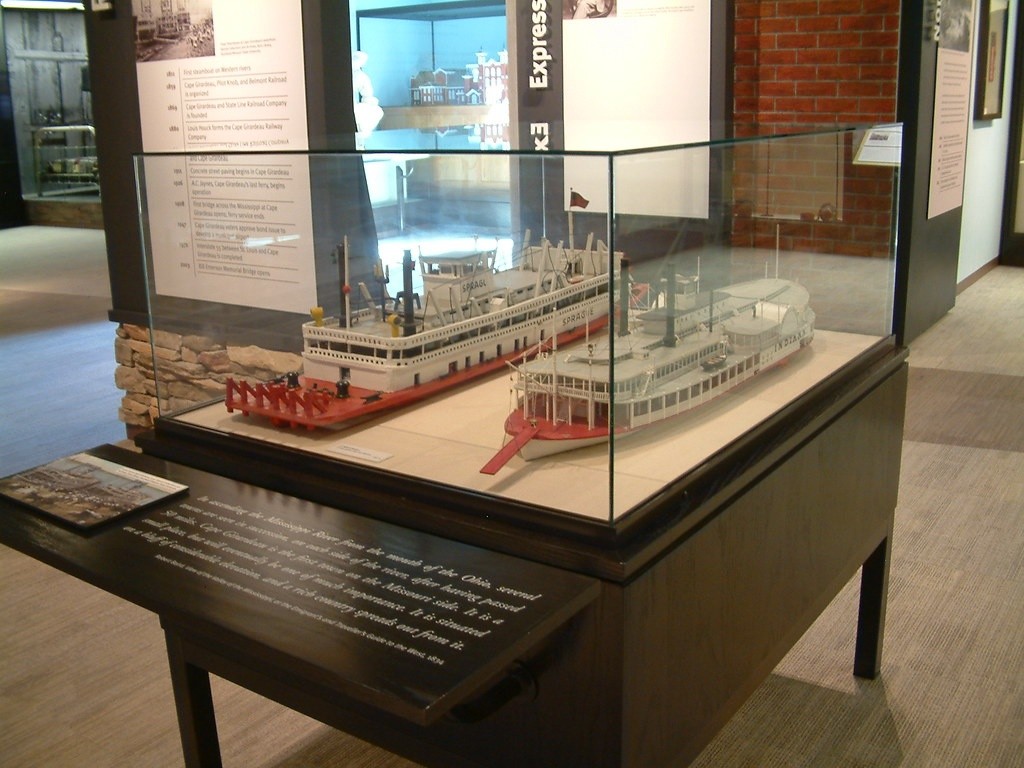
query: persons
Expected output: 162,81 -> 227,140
573,0 -> 616,18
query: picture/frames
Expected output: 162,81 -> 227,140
972,1 -> 1010,122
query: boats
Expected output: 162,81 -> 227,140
481,222 -> 819,478
223,183 -> 653,434
